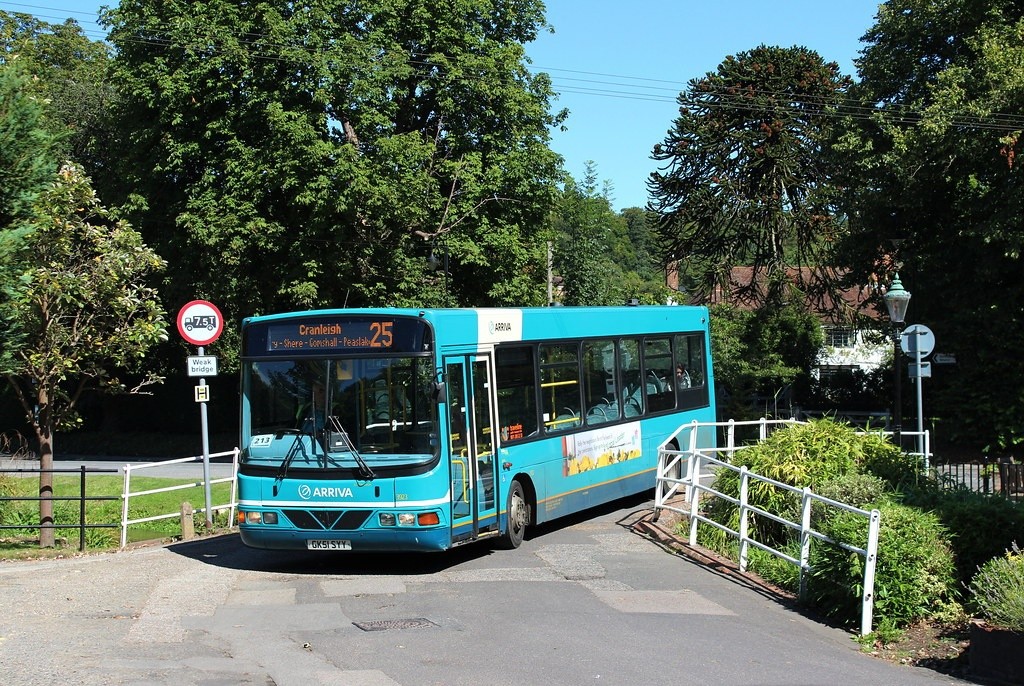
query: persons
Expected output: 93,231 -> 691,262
658,362 -> 688,394
292,381 -> 347,439
620,367 -> 655,417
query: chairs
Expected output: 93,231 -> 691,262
545,368 -> 675,433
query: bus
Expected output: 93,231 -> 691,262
233,299 -> 718,551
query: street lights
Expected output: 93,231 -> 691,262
881,272 -> 908,450
